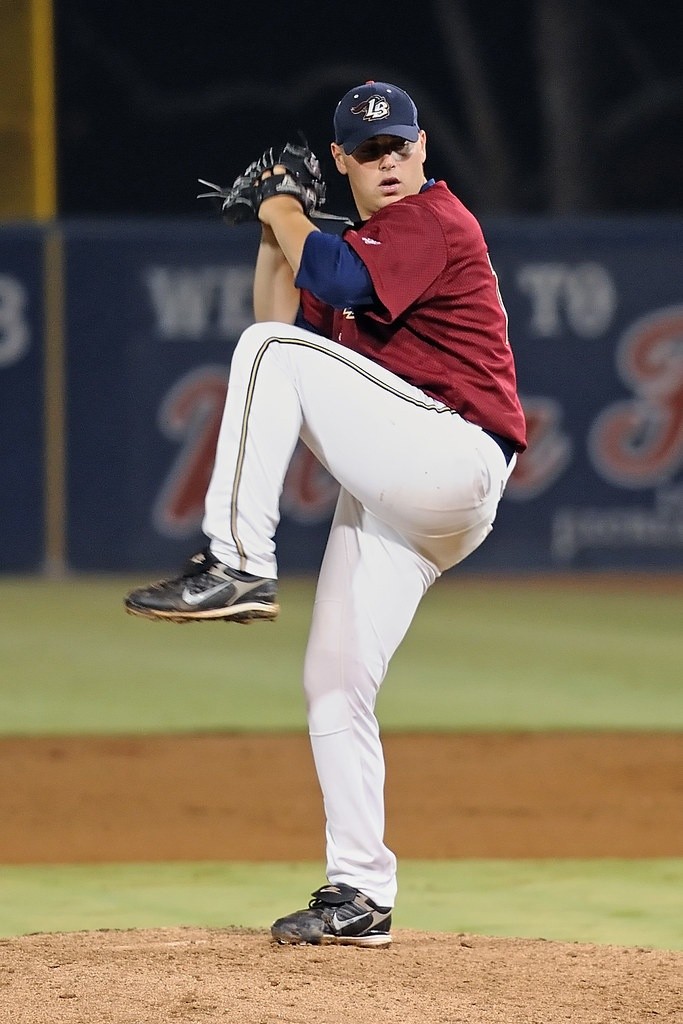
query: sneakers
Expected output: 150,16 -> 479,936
125,545 -> 280,625
271,883 -> 394,947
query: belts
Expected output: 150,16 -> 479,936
483,427 -> 516,465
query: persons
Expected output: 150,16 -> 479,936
123,80 -> 527,943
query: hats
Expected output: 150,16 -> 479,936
334,81 -> 420,155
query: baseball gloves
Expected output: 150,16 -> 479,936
222,143 -> 327,216
